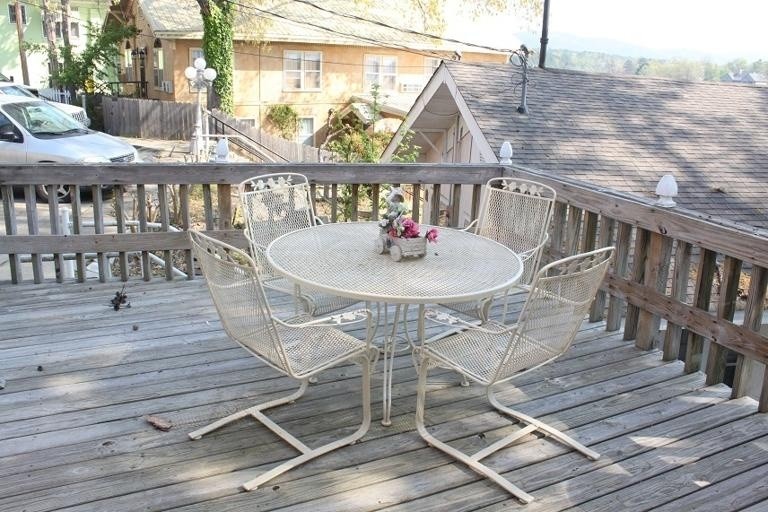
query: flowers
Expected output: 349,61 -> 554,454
379,190 -> 438,244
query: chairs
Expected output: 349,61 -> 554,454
238,172 -> 365,321
186,228 -> 379,491
417,176 -> 557,344
415,246 -> 618,506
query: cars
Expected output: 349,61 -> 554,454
0,96 -> 136,203
0,79 -> 91,130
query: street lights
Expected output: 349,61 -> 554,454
183,56 -> 217,158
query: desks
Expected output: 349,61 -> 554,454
265,221 -> 524,427
